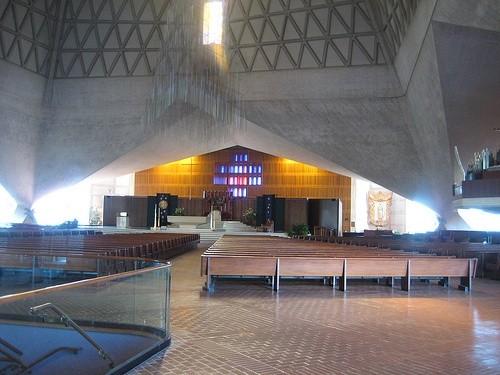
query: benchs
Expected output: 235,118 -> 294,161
0,221 -> 500,289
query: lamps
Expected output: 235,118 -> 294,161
202,0 -> 223,45
